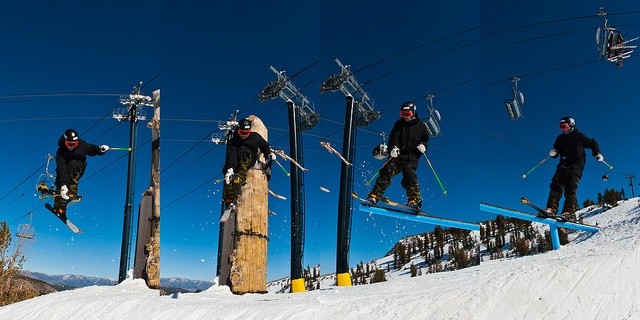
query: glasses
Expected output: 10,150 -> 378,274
400,110 -> 412,118
65,140 -> 78,147
560,124 -> 572,130
237,129 -> 250,136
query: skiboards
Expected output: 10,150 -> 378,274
39,179 -> 83,233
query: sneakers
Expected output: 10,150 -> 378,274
366,194 -> 378,203
545,208 -> 556,215
408,198 -> 421,207
562,212 -> 576,221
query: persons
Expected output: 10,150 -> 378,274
53,129 -> 109,216
222,119 -> 276,212
544,117 -> 604,223
366,102 -> 429,210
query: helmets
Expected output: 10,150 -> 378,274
401,102 -> 416,113
560,117 -> 575,129
238,119 -> 251,129
64,129 -> 78,140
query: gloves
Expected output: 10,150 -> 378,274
417,143 -> 426,153
391,146 -> 400,158
595,153 -> 603,162
61,185 -> 70,200
549,149 -> 556,156
225,168 -> 234,184
101,145 -> 109,152
268,153 -> 276,160
